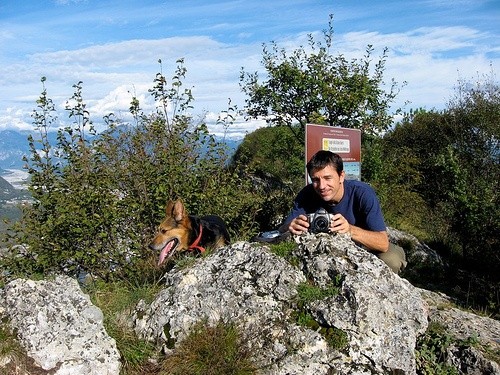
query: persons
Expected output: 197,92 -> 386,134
279,150 -> 406,274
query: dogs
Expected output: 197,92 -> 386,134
149,199 -> 231,265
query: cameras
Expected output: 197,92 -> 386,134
305,208 -> 336,236
